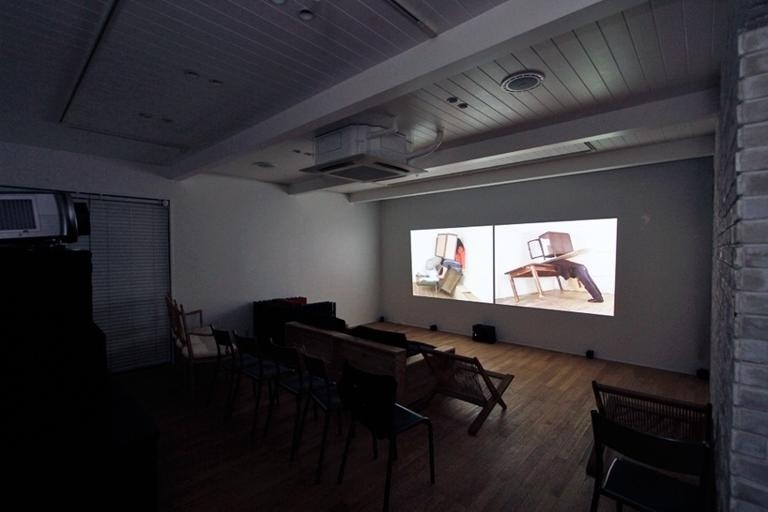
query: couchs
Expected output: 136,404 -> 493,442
286,320 -> 456,434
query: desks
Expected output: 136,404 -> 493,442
503,263 -> 582,303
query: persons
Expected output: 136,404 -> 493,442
550,260 -> 604,303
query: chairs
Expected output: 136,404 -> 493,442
585,379 -> 719,511
418,344 -> 515,436
165,295 -> 436,511
